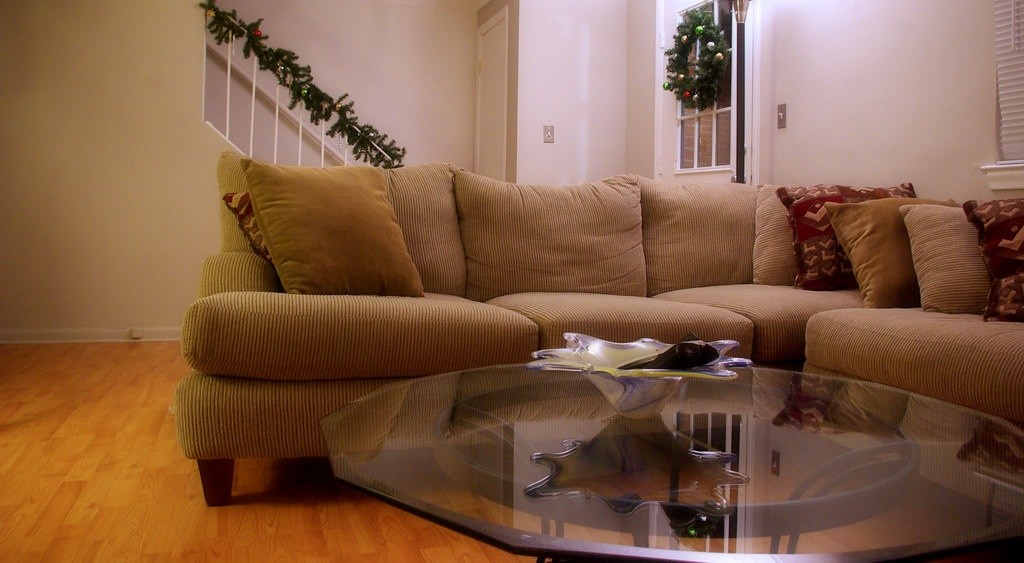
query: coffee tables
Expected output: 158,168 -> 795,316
319,367 -> 1024,563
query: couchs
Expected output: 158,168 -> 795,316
172,162 -> 1024,519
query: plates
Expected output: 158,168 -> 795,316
523,414 -> 752,518
526,333 -> 753,419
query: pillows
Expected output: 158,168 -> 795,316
899,204 -> 991,312
222,192 -> 275,267
825,197 -> 962,309
961,198 -> 1024,322
776,183 -> 917,290
240,158 -> 424,298
752,184 -> 797,286
